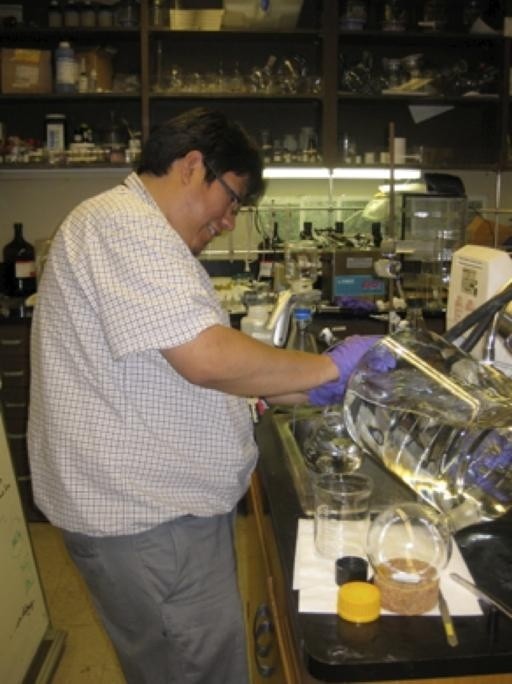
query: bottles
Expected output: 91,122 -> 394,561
336,582 -> 381,646
288,308 -> 317,353
3,222 -> 38,318
53,42 -> 78,92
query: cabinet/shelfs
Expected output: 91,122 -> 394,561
0,313 -> 46,523
0,0 -> 512,177
229,310 -> 448,355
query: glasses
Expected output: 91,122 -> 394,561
203,158 -> 243,215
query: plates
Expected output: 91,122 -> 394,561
271,407 -> 423,518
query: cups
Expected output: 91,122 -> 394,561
312,471 -> 378,562
304,404 -> 362,476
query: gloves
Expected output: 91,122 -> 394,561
327,335 -> 395,386
308,383 -> 346,407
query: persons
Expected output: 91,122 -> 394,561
29,106 -> 397,684
